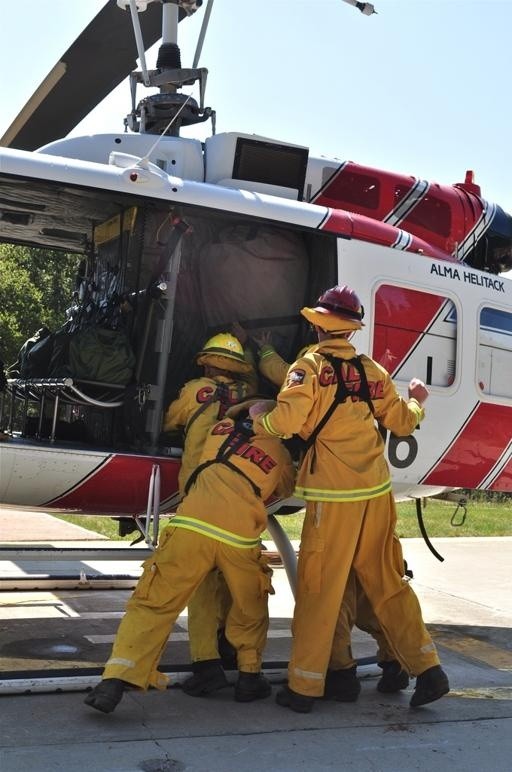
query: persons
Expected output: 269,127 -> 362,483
246,281 -> 452,714
82,392 -> 299,717
154,319 -> 278,703
249,325 -> 415,700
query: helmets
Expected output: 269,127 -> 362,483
195,333 -> 252,367
317,284 -> 361,318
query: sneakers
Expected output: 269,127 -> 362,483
84,678 -> 123,712
324,666 -> 360,702
410,665 -> 450,706
180,627 -> 272,702
277,685 -> 314,713
376,661 -> 409,693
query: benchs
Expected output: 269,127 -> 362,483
5,377 -> 127,444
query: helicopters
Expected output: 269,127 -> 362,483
0,1 -> 512,700
